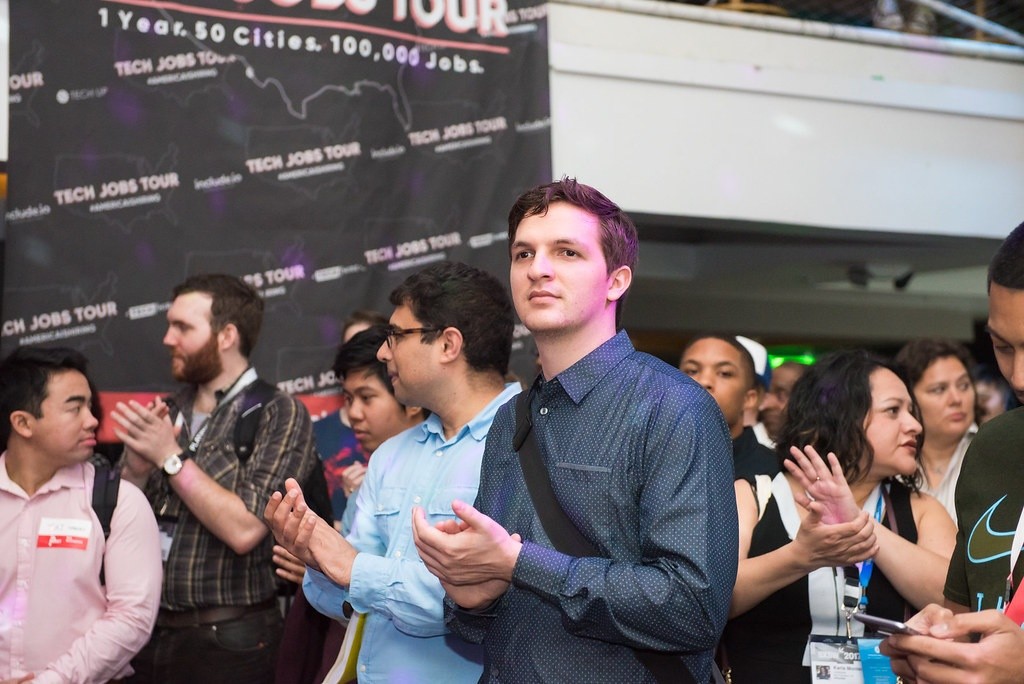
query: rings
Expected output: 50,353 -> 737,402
812,476 -> 820,485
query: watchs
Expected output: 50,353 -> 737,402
160,450 -> 194,476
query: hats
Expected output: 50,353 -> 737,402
734,335 -> 772,392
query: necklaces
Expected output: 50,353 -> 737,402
930,457 -> 948,475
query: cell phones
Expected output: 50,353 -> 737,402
852,613 -> 923,638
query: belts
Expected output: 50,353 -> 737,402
156,596 -> 281,628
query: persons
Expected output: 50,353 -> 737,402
410,175 -> 740,684
678,219 -> 1024,684
0,265 -> 548,684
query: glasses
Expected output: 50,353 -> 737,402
385,324 -> 438,350
